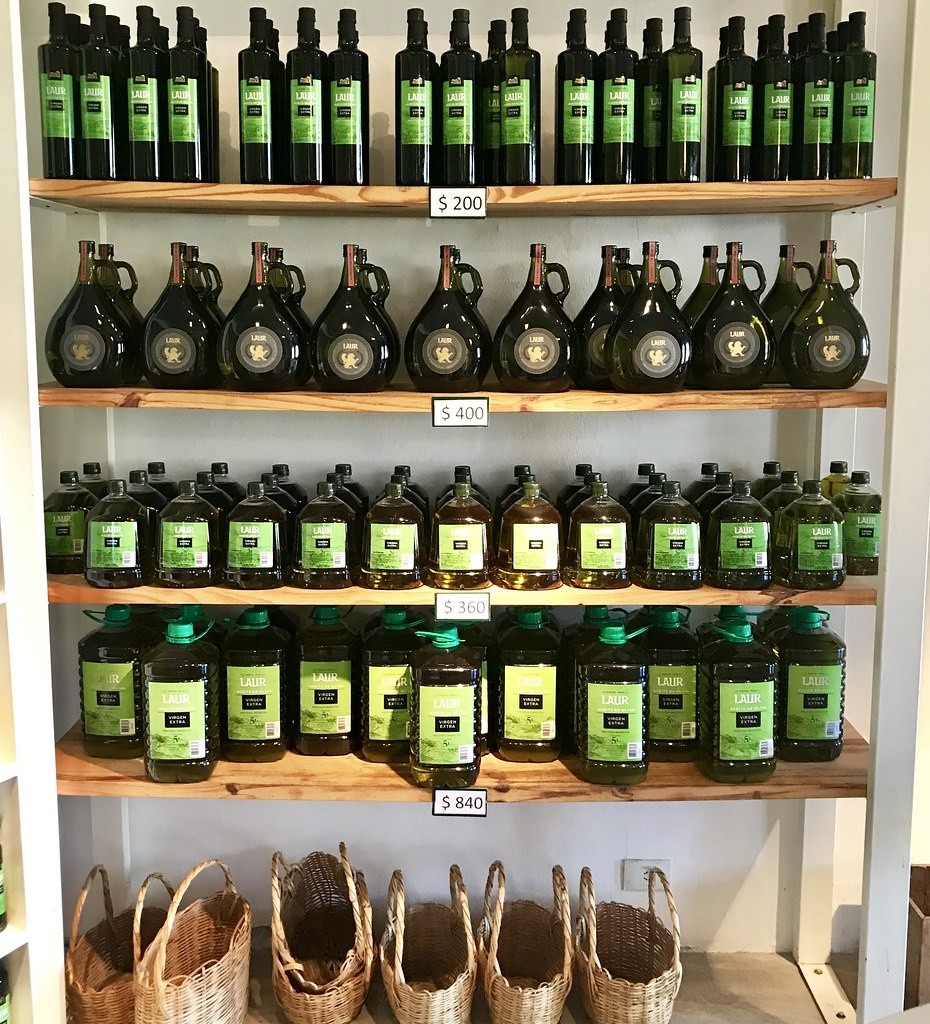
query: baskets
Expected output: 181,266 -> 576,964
377,864 -> 473,1024
573,864 -> 682,1024
66,863 -> 178,1024
475,860 -> 573,1024
134,858 -> 251,1024
270,842 -> 372,1024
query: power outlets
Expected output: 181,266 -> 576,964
623,858 -> 673,892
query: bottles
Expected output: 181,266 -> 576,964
44,240 -> 871,391
77,603 -> 847,784
43,462 -> 883,590
37,2 -> 878,182
0,843 -> 13,1024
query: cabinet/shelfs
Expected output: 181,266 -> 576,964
0,0 -> 930,1024
